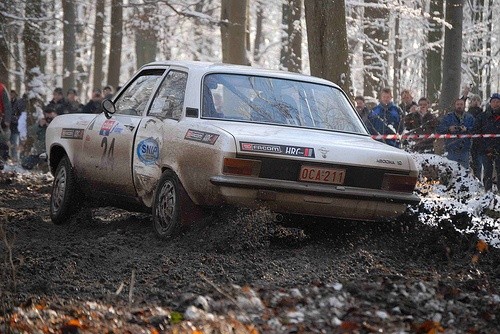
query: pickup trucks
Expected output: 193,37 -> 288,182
46,61 -> 421,241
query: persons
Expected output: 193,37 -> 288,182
354,96 -> 369,125
436,99 -> 475,173
365,88 -> 404,149
0,82 -> 118,169
398,90 -> 417,115
467,94 -> 483,180
400,97 -> 440,154
473,93 -> 500,192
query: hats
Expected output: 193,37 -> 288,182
490,93 -> 500,99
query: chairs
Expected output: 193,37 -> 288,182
203,84 -> 301,124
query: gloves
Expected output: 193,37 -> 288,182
449,125 -> 467,132
